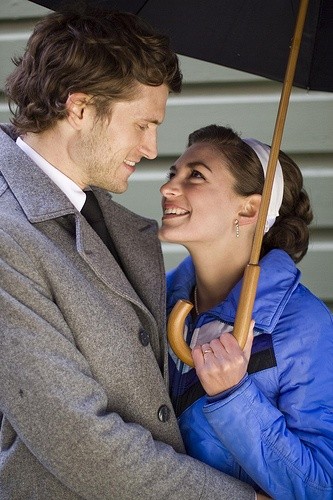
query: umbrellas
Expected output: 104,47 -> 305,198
28,0 -> 333,367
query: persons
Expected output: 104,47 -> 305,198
0,4 -> 272,500
157,124 -> 333,500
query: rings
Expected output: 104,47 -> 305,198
202,349 -> 212,356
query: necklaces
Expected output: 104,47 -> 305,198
194,285 -> 200,316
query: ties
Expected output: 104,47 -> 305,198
83,190 -> 126,269
